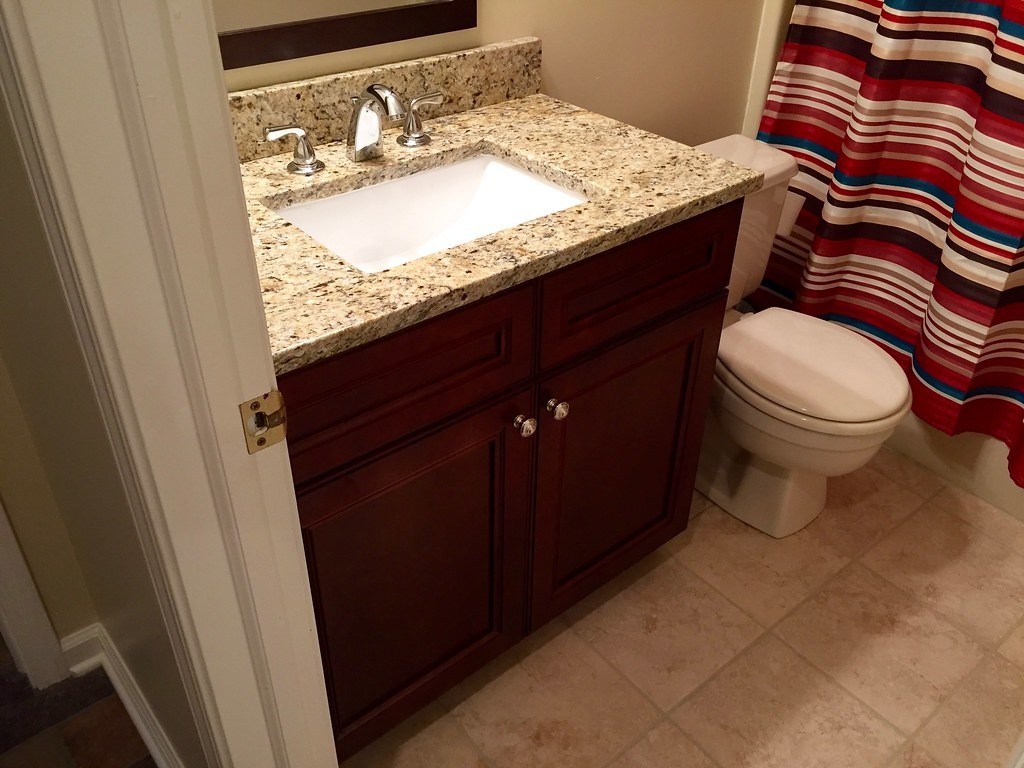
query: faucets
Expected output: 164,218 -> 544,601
345,83 -> 408,163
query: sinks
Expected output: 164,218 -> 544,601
257,137 -> 607,275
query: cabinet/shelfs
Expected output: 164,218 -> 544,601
277,196 -> 746,756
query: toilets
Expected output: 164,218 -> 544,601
691,133 -> 913,540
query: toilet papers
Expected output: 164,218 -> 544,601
775,190 -> 807,238
